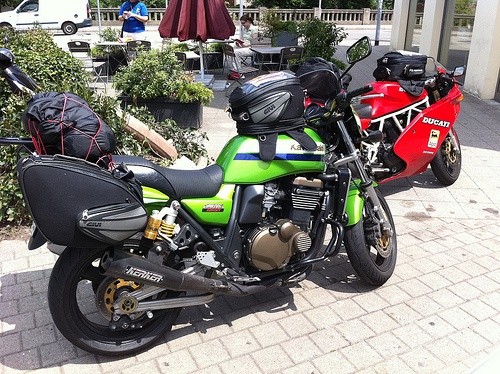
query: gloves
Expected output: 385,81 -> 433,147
303,94 -> 338,127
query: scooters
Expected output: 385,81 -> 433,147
0,43 -> 41,145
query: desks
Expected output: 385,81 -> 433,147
250,47 -> 285,74
181,51 -> 200,73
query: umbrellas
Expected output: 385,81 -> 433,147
158,1 -> 236,79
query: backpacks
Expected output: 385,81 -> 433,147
22,91 -> 116,170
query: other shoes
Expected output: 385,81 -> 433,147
228,73 -> 245,80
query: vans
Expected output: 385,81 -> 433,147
0,0 -> 93,33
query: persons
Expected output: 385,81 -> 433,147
231,15 -> 257,70
118,0 -> 148,40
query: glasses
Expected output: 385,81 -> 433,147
241,21 -> 246,24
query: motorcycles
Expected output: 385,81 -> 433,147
15,36 -> 397,359
297,49 -> 465,186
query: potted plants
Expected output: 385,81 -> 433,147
116,49 -> 213,130
257,7 -> 299,47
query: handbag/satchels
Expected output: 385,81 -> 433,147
373,52 -> 427,96
225,70 -> 317,162
120,23 -> 124,38
291,56 -> 342,99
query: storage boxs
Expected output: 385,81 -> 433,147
19,155 -> 148,248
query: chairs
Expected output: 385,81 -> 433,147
67,40 -> 107,95
192,40 -> 303,97
127,40 -> 187,72
